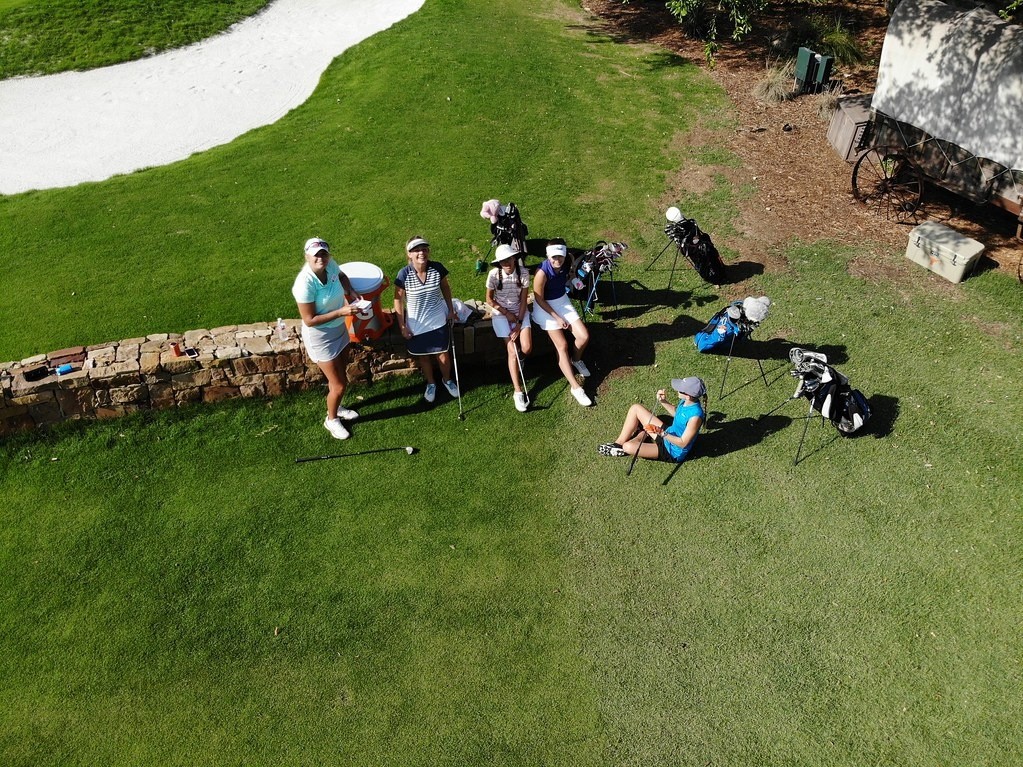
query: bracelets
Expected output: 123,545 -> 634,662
661,431 -> 667,437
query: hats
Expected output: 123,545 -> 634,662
406,239 -> 430,252
490,244 -> 522,266
671,376 -> 705,398
546,244 -> 567,258
304,238 -> 330,256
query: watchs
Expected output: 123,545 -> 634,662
517,320 -> 523,324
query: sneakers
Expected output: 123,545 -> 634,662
324,416 -> 350,440
442,379 -> 458,397
570,387 -> 592,406
570,358 -> 590,377
597,442 -> 626,456
423,384 -> 436,402
514,392 -> 527,412
336,406 -> 359,420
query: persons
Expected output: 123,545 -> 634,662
531,237 -> 593,407
596,376 -> 706,462
291,238 -> 364,440
394,235 -> 461,403
485,243 -> 534,412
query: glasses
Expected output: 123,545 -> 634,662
414,248 -> 428,252
306,242 -> 329,251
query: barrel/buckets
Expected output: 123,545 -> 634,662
338,262 -> 393,343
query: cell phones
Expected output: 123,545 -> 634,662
185,348 -> 198,358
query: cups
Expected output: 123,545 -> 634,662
169,342 -> 181,357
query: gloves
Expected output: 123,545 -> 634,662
643,424 -> 664,435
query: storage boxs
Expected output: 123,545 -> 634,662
905,221 -> 984,284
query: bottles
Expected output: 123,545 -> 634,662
278,318 -> 288,341
476,259 -> 482,273
578,269 -> 586,278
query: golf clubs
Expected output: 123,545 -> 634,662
663,224 -> 692,254
476,202 -> 515,276
730,324 -> 761,341
581,241 -> 629,320
295,446 -> 414,464
506,316 -> 531,408
450,324 -> 466,422
789,368 -> 821,398
626,389 -> 666,476
